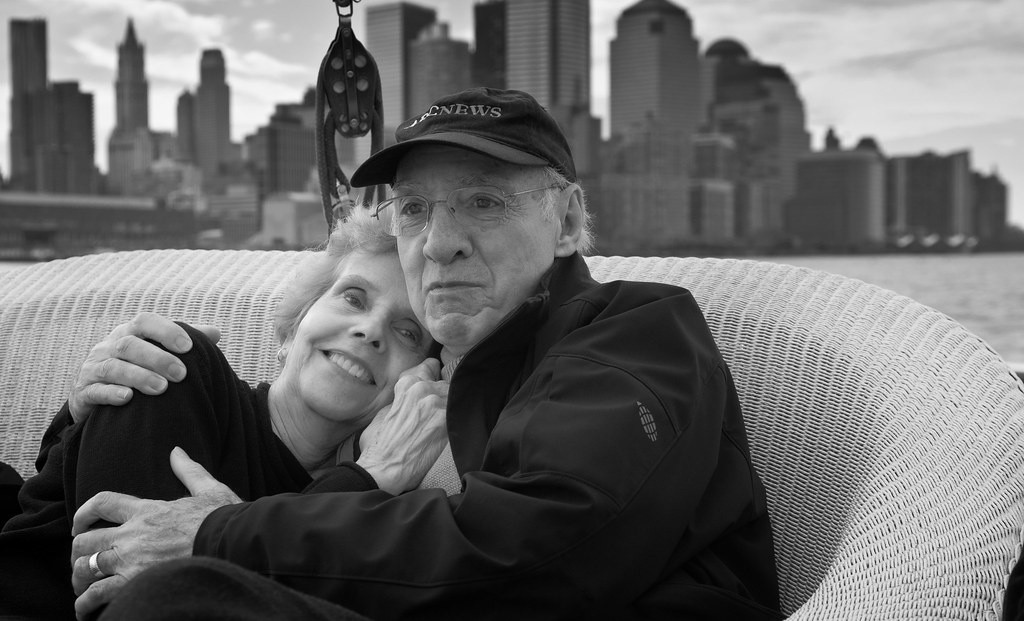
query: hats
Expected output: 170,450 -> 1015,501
348,86 -> 578,184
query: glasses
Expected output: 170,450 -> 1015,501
369,184 -> 564,239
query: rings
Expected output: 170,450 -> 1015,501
89,552 -> 105,578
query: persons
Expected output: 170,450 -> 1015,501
0,188 -> 453,621
38,86 -> 784,621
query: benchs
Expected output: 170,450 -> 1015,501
0,247 -> 1024,621
